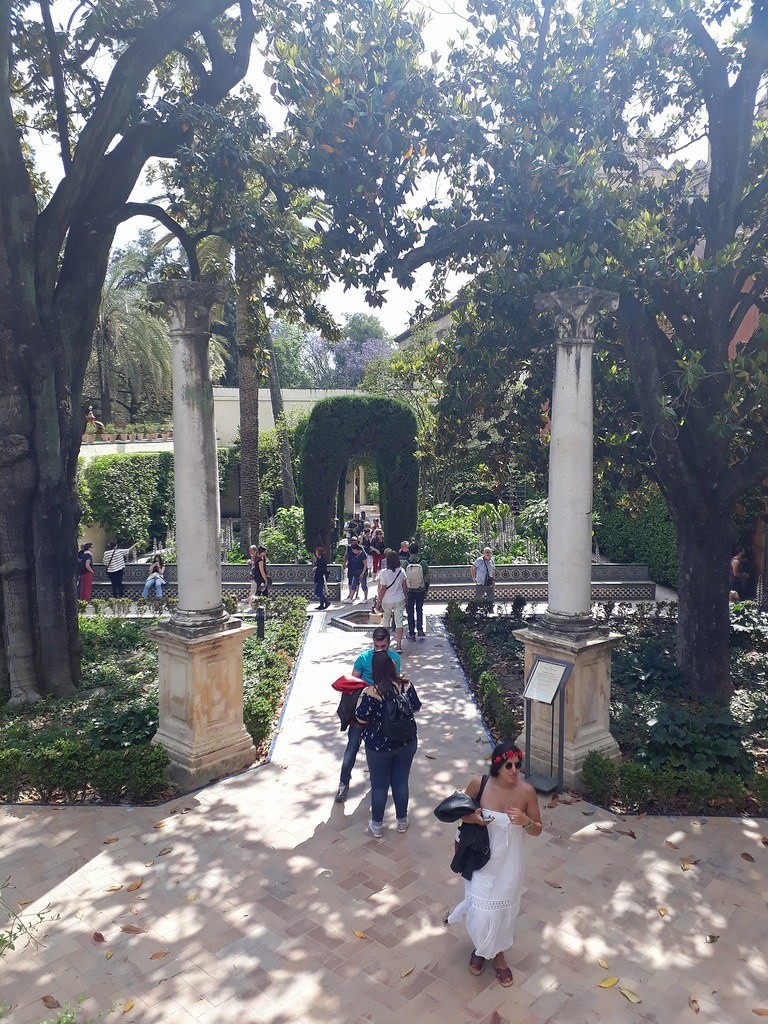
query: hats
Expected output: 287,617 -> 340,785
258,546 -> 267,554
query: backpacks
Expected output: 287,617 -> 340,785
377,679 -> 416,737
406,559 -> 424,589
77,558 -> 87,574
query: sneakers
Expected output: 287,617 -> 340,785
367,820 -> 382,838
397,817 -> 409,832
336,782 -> 349,802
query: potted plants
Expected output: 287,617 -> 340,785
369,595 -> 382,623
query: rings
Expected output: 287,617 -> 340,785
511,817 -> 514,819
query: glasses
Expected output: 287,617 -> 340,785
351,539 -> 357,542
501,762 -> 522,770
378,533 -> 382,535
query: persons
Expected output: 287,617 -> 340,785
447,742 -> 542,987
246,544 -> 270,603
334,627 -> 402,801
103,540 -> 141,599
344,511 -> 385,582
312,546 -> 331,610
729,546 -> 750,600
355,650 -> 419,837
397,540 -> 412,560
378,547 -> 397,631
76,542 -> 94,603
403,547 -> 431,642
143,558 -> 167,600
376,552 -> 408,655
342,536 -> 369,604
470,547 -> 496,615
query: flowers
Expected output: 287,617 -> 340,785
493,750 -> 524,764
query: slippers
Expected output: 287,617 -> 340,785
493,962 -> 514,987
469,949 -> 485,975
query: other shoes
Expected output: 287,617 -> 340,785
417,631 -> 426,638
405,632 -> 415,641
315,605 -> 324,609
343,599 -> 352,604
396,647 -> 402,652
361,599 -> 368,603
484,574 -> 494,586
325,603 -> 331,608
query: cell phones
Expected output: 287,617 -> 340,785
483,817 -> 493,822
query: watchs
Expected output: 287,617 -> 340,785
521,819 -> 534,832
